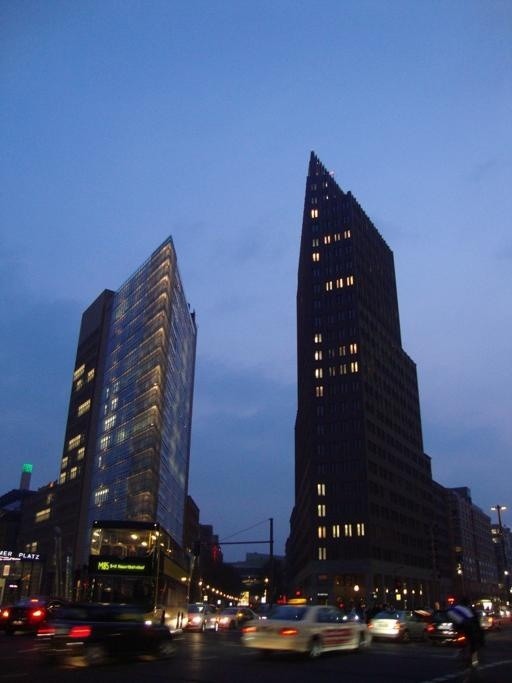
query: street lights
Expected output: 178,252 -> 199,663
491,504 -> 511,586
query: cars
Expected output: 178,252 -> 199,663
427,616 -> 468,646
239,604 -> 373,660
475,608 -> 512,632
0,590 -> 259,673
367,609 -> 430,644
